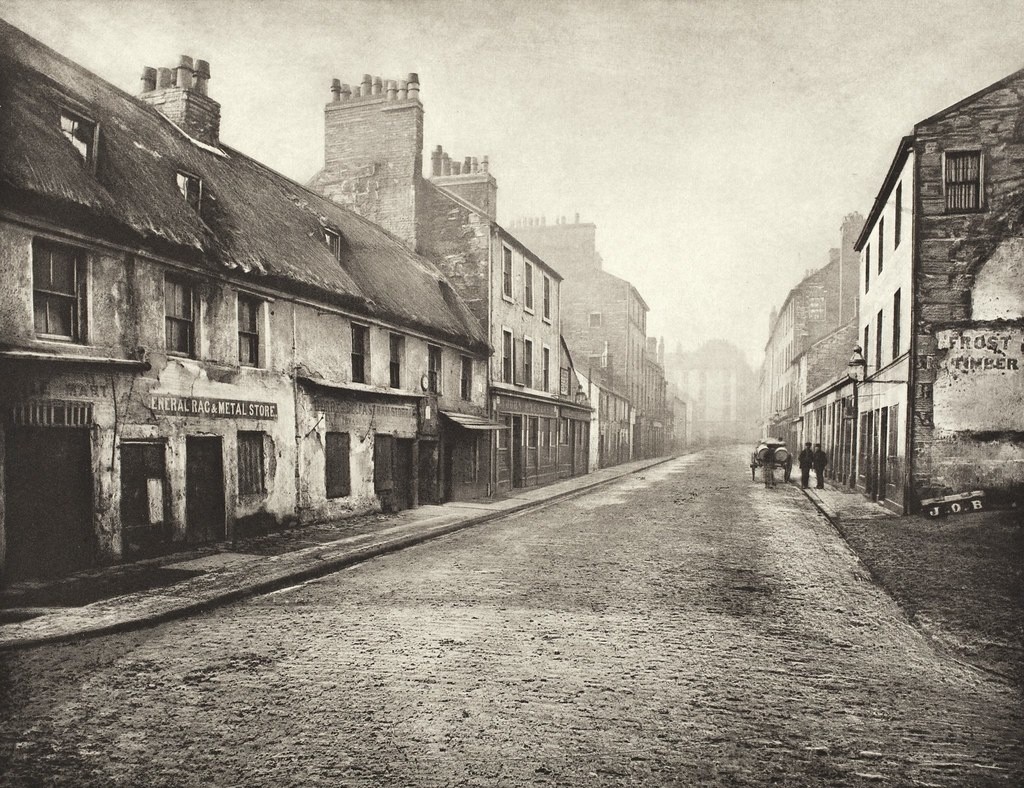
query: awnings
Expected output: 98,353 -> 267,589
298,376 -> 427,401
0,348 -> 154,373
440,411 -> 512,434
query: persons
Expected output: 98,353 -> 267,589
798,442 -> 814,490
763,447 -> 776,490
812,443 -> 828,490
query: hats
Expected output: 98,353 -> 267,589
815,444 -> 821,447
805,442 -> 811,446
